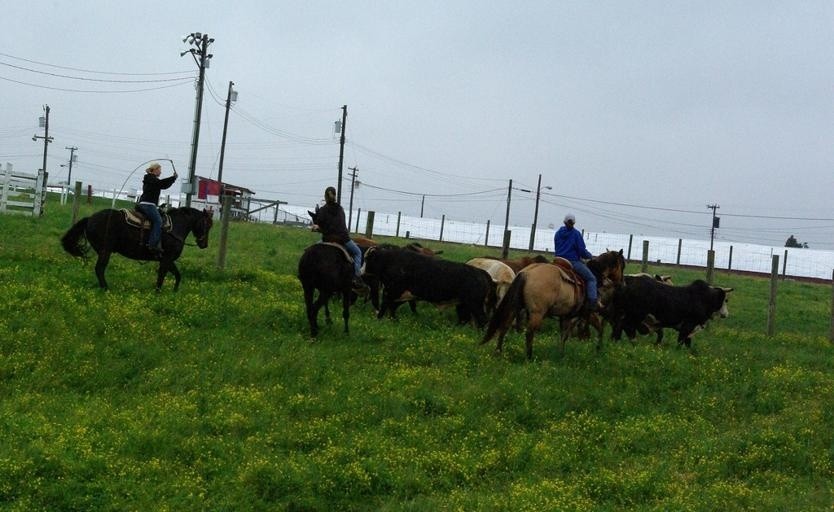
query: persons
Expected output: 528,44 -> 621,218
553,212 -> 600,309
313,186 -> 366,288
137,161 -> 178,254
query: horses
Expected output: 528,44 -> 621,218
298,204 -> 364,344
478,249 -> 627,359
60,206 -> 213,291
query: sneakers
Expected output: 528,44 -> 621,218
587,301 -> 606,310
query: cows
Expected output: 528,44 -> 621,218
575,272 -> 734,351
331,236 -> 553,334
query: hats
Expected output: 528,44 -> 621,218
565,215 -> 575,223
